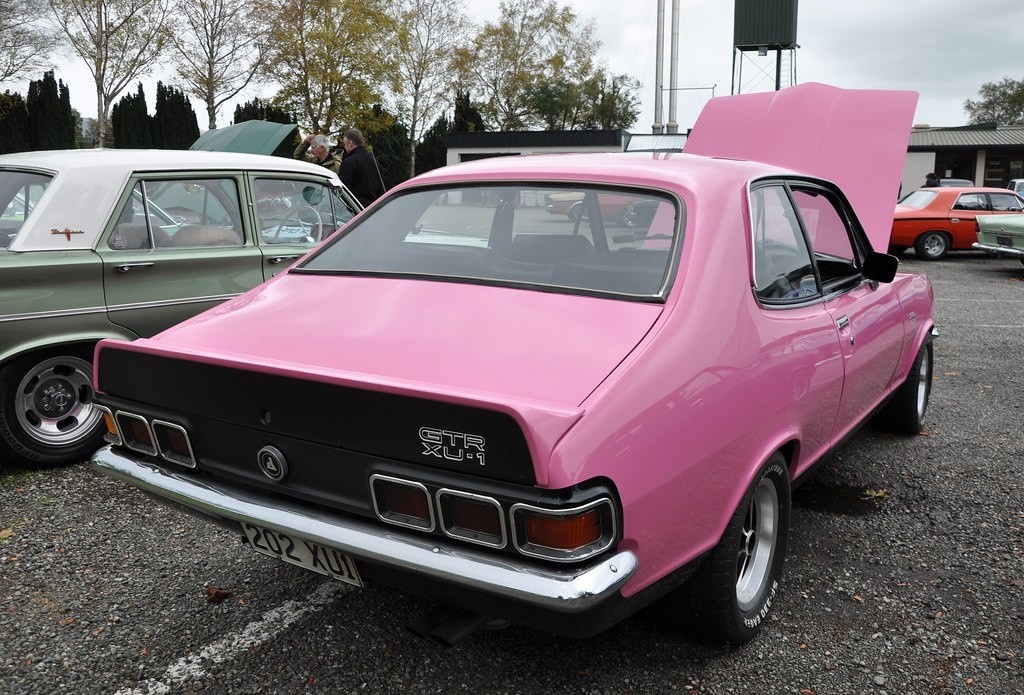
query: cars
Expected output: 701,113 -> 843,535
888,187 -> 1024,260
1008,179 -> 1024,201
0,119 -> 366,466
972,212 -> 1024,263
545,190 -> 659,228
86,81 -> 937,650
940,179 -> 974,187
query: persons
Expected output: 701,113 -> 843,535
294,133 -> 343,175
340,129 -> 386,208
921,173 -> 941,188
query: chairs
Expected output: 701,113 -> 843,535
115,223 -> 173,249
511,234 -> 598,262
169,223 -> 242,246
